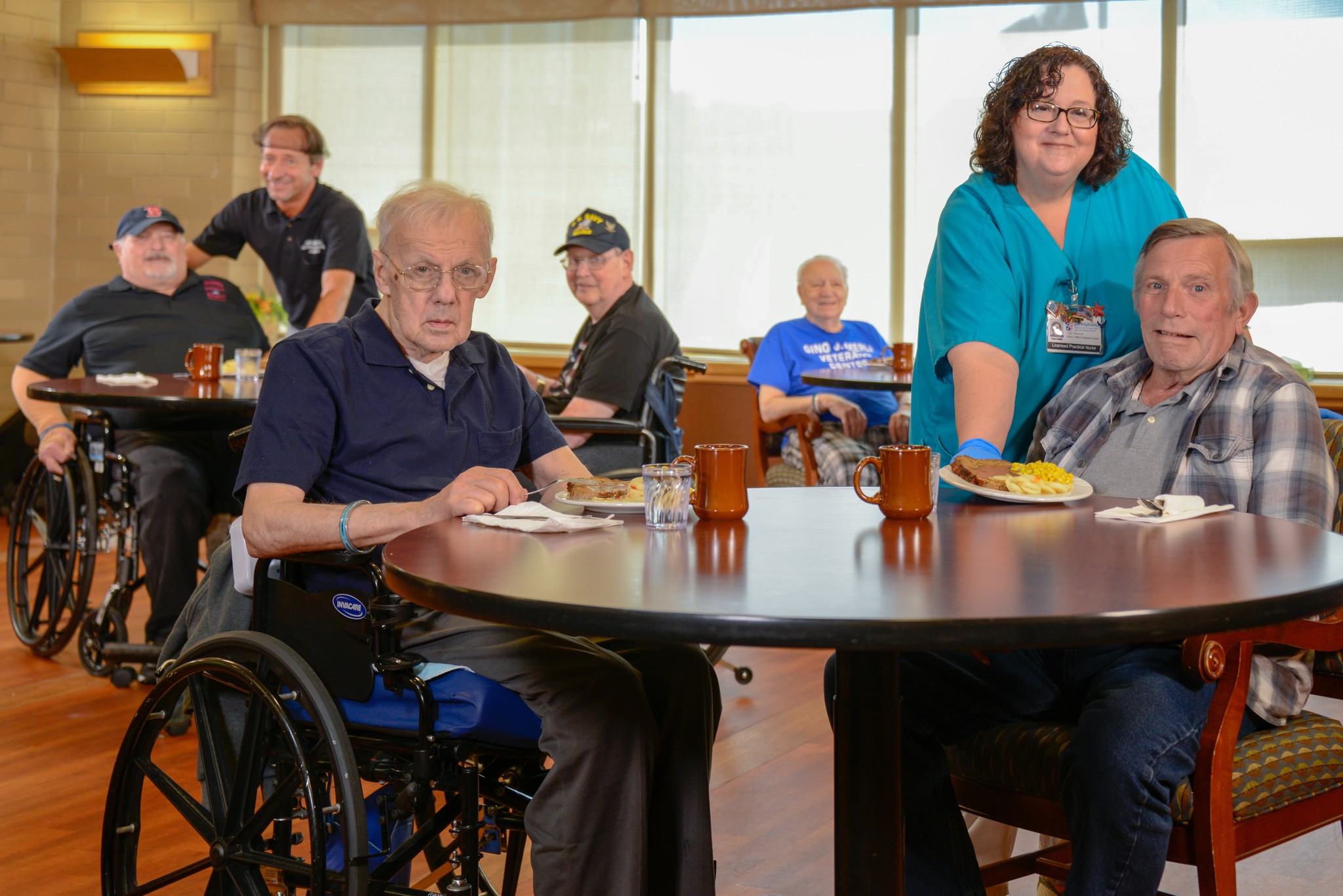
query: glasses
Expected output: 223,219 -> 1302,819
561,251 -> 625,271
378,250 -> 491,290
1025,100 -> 1101,130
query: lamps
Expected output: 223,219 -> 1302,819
53,26 -> 222,104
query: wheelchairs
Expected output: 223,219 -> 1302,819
544,353 -> 707,484
98,519 -> 557,895
0,329 -> 215,689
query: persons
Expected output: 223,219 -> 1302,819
511,208 -> 685,481
13,206 -> 273,738
744,255 -> 913,486
183,114 -> 381,329
910,42 -> 1253,464
154,173 -> 722,896
827,219 -> 1339,896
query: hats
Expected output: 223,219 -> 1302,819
116,205 -> 185,239
553,208 -> 630,256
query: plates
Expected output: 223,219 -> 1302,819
554,490 -> 645,514
862,364 -> 892,370
939,464 -> 1093,503
221,373 -> 263,380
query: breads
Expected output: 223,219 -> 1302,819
949,454 -> 1021,491
567,476 -> 630,500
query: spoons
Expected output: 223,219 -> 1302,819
1137,499 -> 1163,510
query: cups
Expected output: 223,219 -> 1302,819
881,342 -> 913,372
184,343 -> 225,383
672,444 -> 749,521
234,348 -> 262,382
642,463 -> 692,530
931,452 -> 941,510
853,445 -> 933,519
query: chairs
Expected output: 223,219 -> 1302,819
739,334 -> 823,487
988,376 -> 1343,896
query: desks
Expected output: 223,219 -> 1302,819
24,363 -> 279,431
377,482 -> 1343,896
799,361 -> 917,393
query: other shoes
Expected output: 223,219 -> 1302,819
137,638 -> 164,686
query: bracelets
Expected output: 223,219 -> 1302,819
38,422 -> 77,441
536,373 -> 546,396
339,499 -> 377,555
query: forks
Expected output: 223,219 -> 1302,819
1130,513 -> 1160,517
524,478 -> 598,495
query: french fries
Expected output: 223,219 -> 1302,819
990,474 -> 1071,495
593,477 -> 647,502
